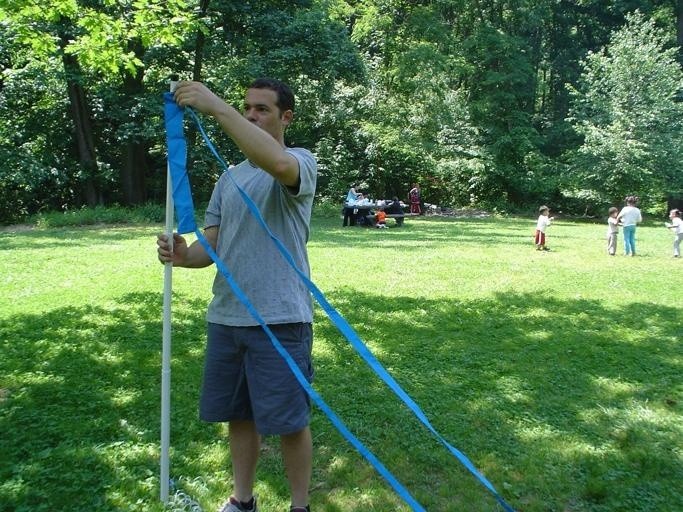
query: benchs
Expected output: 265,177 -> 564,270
365,212 -> 411,225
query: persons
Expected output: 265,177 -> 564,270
342,180 -> 423,229
616,195 -> 643,256
606,207 -> 619,255
156,75 -> 320,510
535,204 -> 555,251
665,208 -> 683,257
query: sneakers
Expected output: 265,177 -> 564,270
290,504 -> 310,512
219,494 -> 256,512
536,247 -> 550,251
